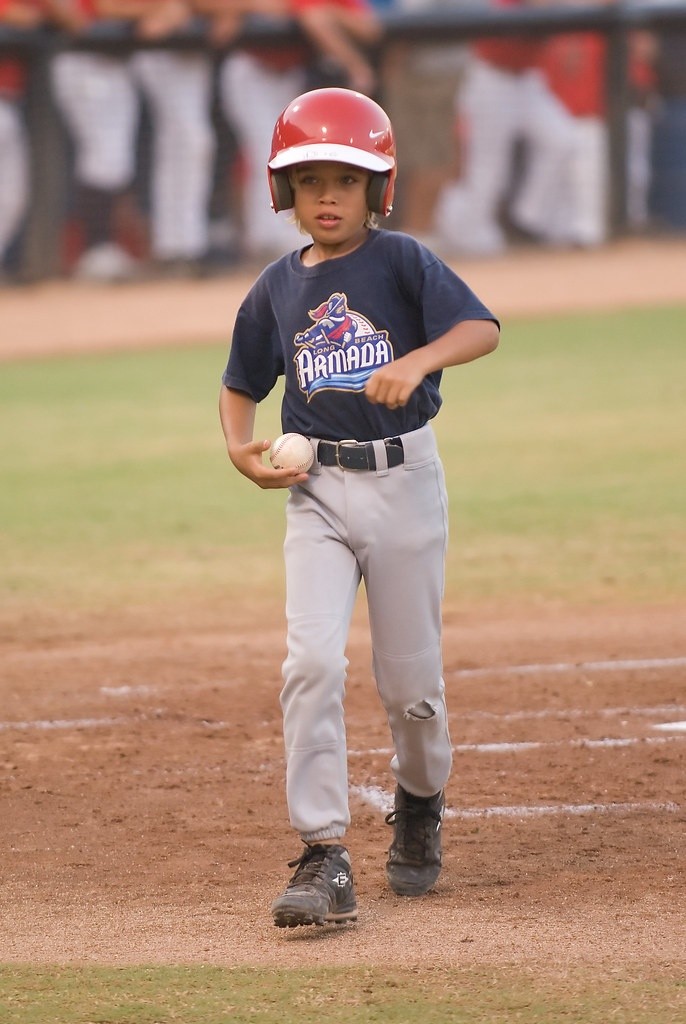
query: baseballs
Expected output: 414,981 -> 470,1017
269,432 -> 314,475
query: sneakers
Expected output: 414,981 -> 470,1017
271,838 -> 359,928
385,782 -> 447,895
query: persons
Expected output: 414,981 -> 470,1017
0,1 -> 662,286
219,88 -> 501,926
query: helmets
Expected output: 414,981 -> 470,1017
267,88 -> 397,216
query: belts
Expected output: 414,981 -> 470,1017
306,436 -> 405,471
